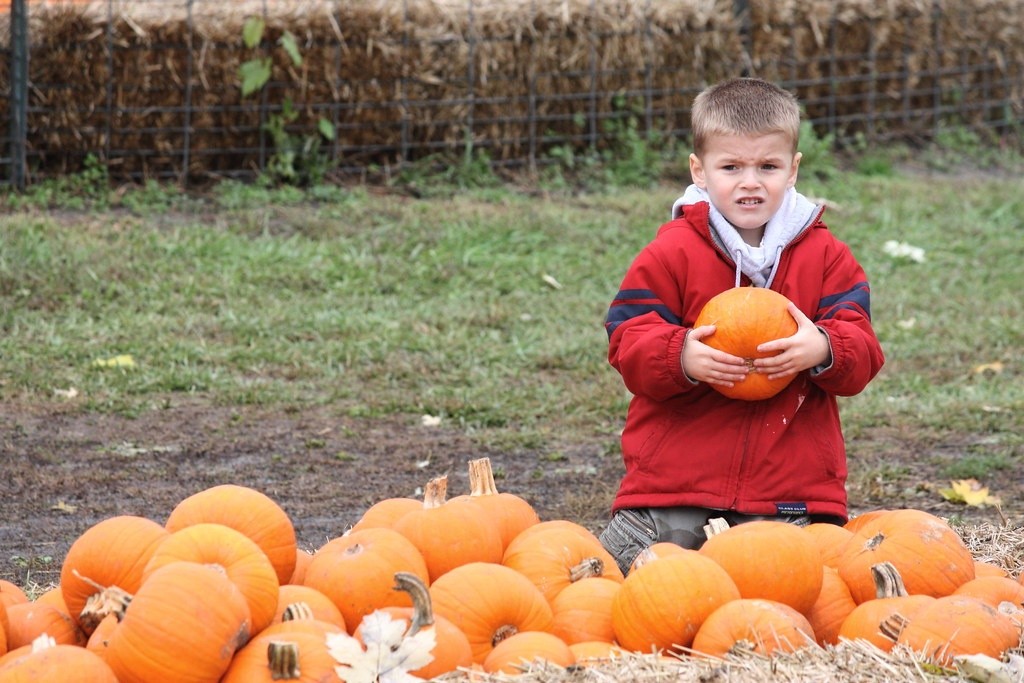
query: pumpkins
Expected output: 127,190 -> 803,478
692,287 -> 801,401
0,455 -> 1024,683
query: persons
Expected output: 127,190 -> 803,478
598,78 -> 884,577
